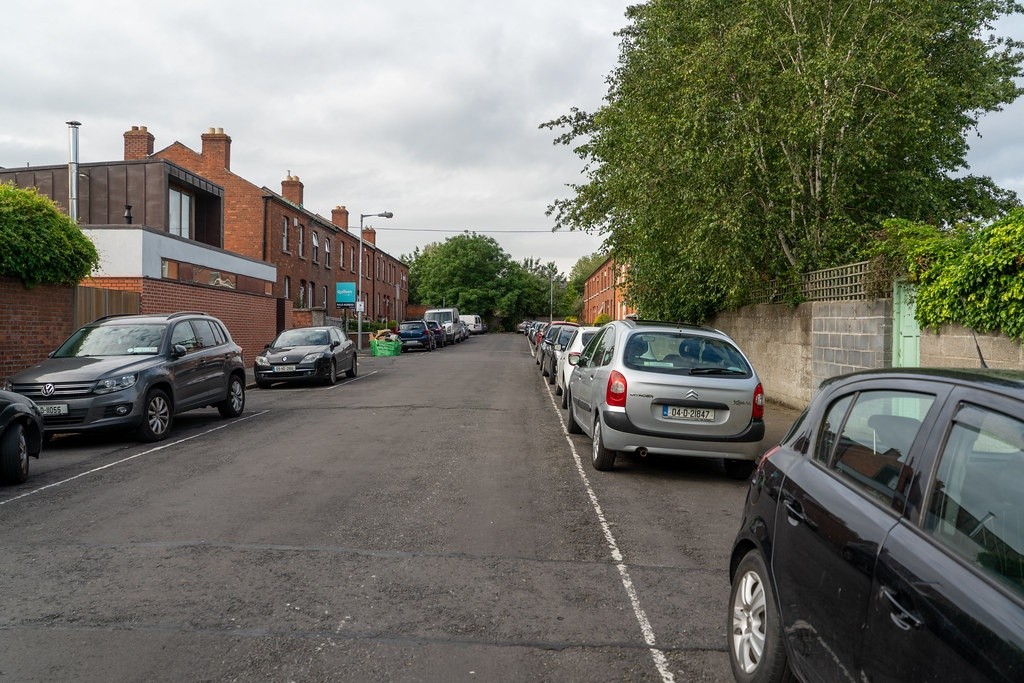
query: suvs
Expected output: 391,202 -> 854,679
396,319 -> 436,353
1,311 -> 247,445
426,318 -> 447,348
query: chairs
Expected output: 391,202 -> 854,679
869,415 -> 924,495
678,338 -> 723,367
631,338 -> 648,361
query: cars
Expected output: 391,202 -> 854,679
254,326 -> 357,389
459,320 -> 470,342
725,364 -> 1023,683
482,323 -> 489,334
0,390 -> 45,487
553,327 -> 606,409
516,319 -> 582,385
567,319 -> 766,480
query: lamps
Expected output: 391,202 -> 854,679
123,205 -> 134,224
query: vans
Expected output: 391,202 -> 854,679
459,314 -> 483,335
422,307 -> 462,345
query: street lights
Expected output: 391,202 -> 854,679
358,211 -> 393,352
